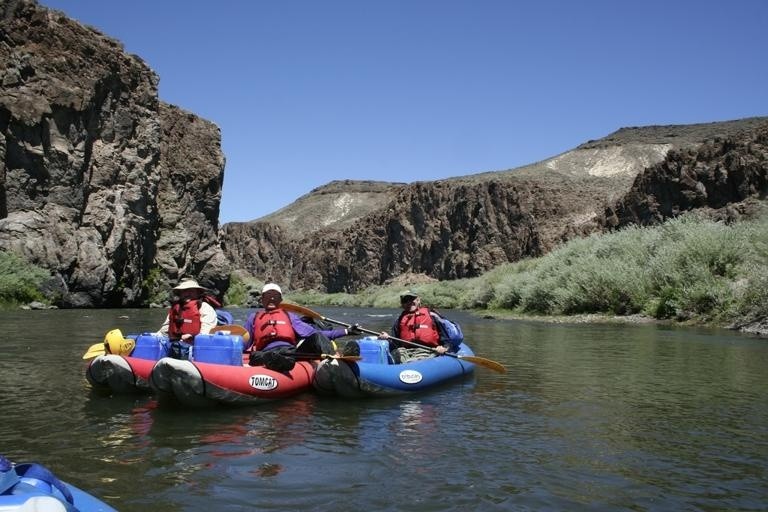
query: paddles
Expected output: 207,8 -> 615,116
278,301 -> 509,373
81,343 -> 106,361
281,351 -> 362,362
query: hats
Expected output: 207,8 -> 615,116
400,288 -> 418,297
262,283 -> 282,296
172,280 -> 206,296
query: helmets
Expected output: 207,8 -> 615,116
104,329 -> 136,356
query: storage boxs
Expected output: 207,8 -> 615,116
193,333 -> 244,367
357,338 -> 390,366
125,333 -> 172,361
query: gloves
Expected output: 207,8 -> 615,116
348,323 -> 364,335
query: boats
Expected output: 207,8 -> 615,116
84,344 -> 341,405
312,334 -> 477,398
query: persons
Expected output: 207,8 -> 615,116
156,278 -> 218,338
243,282 -> 364,372
376,287 -> 454,365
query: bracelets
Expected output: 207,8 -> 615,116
345,328 -> 349,335
